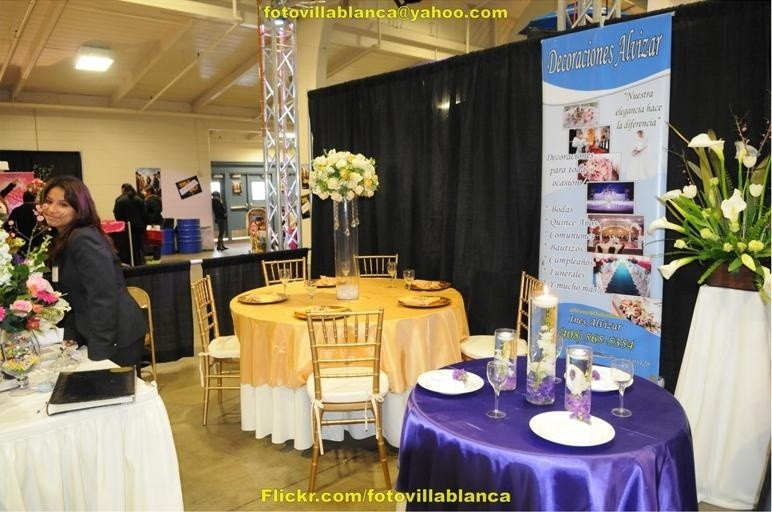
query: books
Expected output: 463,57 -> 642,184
47,363 -> 137,416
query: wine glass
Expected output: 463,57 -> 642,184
388,263 -> 400,289
277,268 -> 291,298
608,356 -> 635,420
304,278 -> 317,304
402,269 -> 416,292
485,359 -> 510,421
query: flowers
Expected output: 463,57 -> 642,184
0,219 -> 70,332
309,149 -> 379,203
646,115 -> 770,301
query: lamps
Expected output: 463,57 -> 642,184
74,46 -> 114,72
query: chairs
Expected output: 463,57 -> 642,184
261,257 -> 306,286
190,275 -> 241,427
126,286 -> 156,382
460,271 -> 547,361
353,254 -> 399,278
305,309 -> 393,492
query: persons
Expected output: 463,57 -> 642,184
4,191 -> 58,258
572,129 -> 587,153
136,171 -> 162,198
41,175 -> 149,378
632,129 -> 648,158
113,183 -> 164,266
212,191 -> 229,250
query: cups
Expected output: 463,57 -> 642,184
492,328 -> 518,393
146,224 -> 161,231
564,344 -> 594,415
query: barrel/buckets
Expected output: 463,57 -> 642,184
177,217 -> 202,254
160,217 -> 175,256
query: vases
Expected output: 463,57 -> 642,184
333,198 -> 360,300
703,257 -> 756,292
2,330 -> 38,397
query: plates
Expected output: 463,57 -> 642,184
234,292 -> 288,304
312,278 -> 341,287
296,304 -> 354,321
564,365 -> 633,392
528,410 -> 616,448
562,106 -> 599,128
399,295 -> 450,308
405,279 -> 452,291
416,369 -> 485,395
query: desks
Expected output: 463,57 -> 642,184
1,358 -> 184,512
